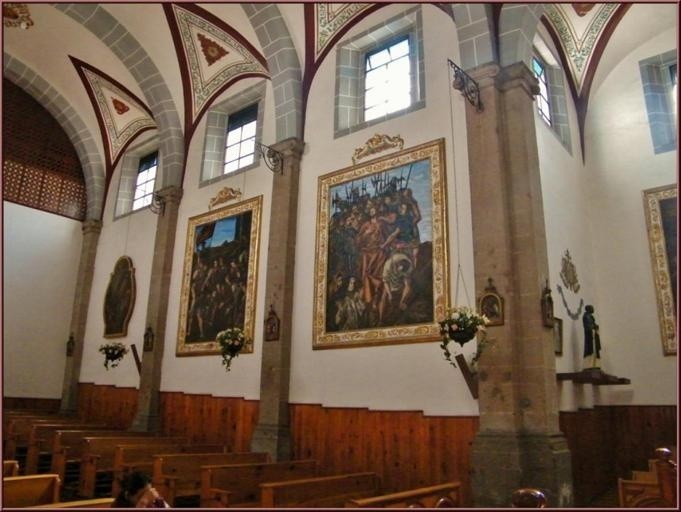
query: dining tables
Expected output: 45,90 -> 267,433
99,343 -> 129,370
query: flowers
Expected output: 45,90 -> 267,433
439,305 -> 491,370
216,327 -> 247,371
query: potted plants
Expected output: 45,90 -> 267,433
642,183 -> 677,358
263,303 -> 279,341
540,287 -> 553,328
99,343 -> 129,370
477,286 -> 504,326
312,136 -> 448,352
175,194 -> 265,357
552,316 -> 563,357
102,255 -> 136,339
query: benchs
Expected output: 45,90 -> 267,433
0,405 -> 465,508
618,447 -> 678,506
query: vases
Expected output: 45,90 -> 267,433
224,343 -> 244,354
448,327 -> 475,346
216,327 -> 247,371
439,305 -> 491,370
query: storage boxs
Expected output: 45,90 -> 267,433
618,447 -> 678,506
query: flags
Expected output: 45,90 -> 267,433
224,343 -> 244,354
448,327 -> 475,346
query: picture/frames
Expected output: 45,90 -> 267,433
540,287 -> 553,328
263,303 -> 279,341
102,255 -> 136,339
175,194 -> 265,357
552,316 -> 563,357
477,286 -> 504,326
312,136 -> 448,352
642,183 -> 677,358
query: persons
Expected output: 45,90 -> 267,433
109,471 -> 171,509
581,304 -> 602,369
327,184 -> 432,331
183,256 -> 246,341
